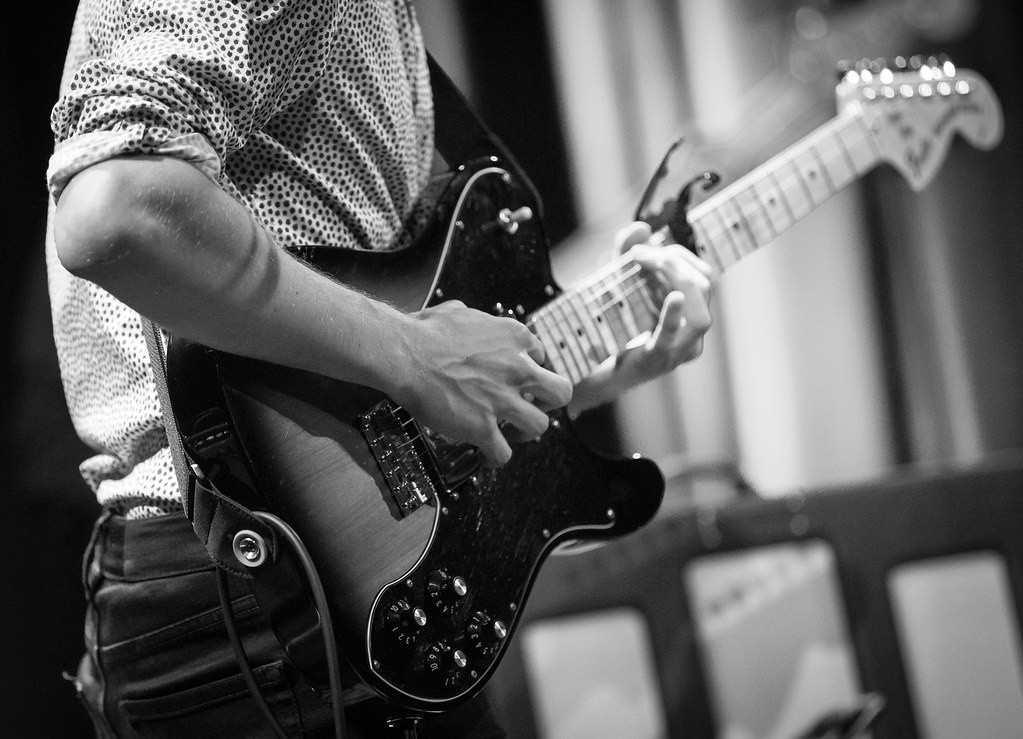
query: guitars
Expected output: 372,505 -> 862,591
166,53 -> 1004,714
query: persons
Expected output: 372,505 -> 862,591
43,0 -> 723,739
